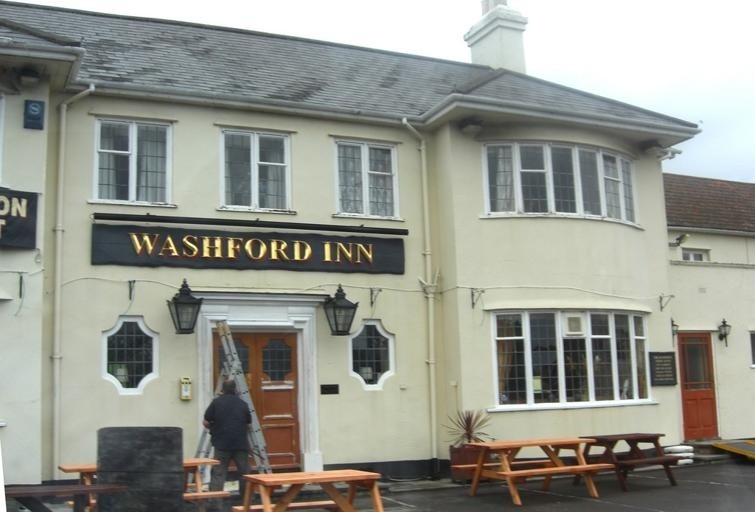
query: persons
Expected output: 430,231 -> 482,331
202,379 -> 252,512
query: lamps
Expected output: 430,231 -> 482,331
670,234 -> 689,247
671,319 -> 678,345
718,318 -> 732,347
166,279 -> 203,334
323,284 -> 359,336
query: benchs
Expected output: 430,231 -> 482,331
451,459 -> 550,471
232,502 -> 336,512
496,464 -> 614,476
615,456 -> 688,468
66,490 -> 229,507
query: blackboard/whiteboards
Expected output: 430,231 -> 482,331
649,351 -> 677,386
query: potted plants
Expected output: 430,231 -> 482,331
440,409 -> 496,486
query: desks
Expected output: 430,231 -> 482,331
58,457 -> 221,512
4,484 -> 127,512
572,432 -> 678,492
243,469 -> 384,512
464,438 -> 599,505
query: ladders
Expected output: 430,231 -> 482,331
186,320 -> 272,484
